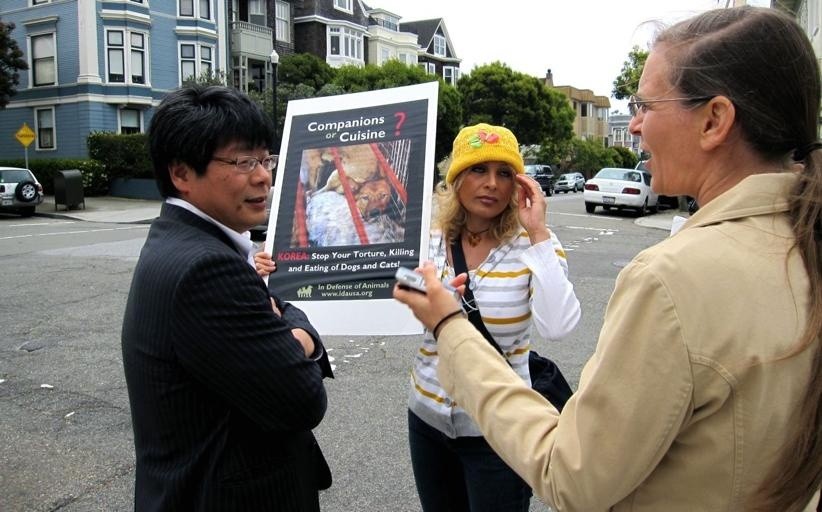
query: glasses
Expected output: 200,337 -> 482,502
628,94 -> 715,117
208,152 -> 278,171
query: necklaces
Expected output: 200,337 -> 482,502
462,225 -> 489,247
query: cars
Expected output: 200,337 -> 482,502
584,161 -> 679,216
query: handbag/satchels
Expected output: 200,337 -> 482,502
530,350 -> 573,414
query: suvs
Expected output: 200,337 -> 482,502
0,166 -> 43,218
523,164 -> 585,195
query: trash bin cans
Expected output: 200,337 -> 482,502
54,169 -> 85,211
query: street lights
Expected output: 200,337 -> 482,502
269,49 -> 280,144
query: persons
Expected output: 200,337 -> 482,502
253,123 -> 581,512
394,4 -> 822,511
120,75 -> 334,511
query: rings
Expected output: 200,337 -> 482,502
538,186 -> 543,193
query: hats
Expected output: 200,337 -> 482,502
446,123 -> 525,189
